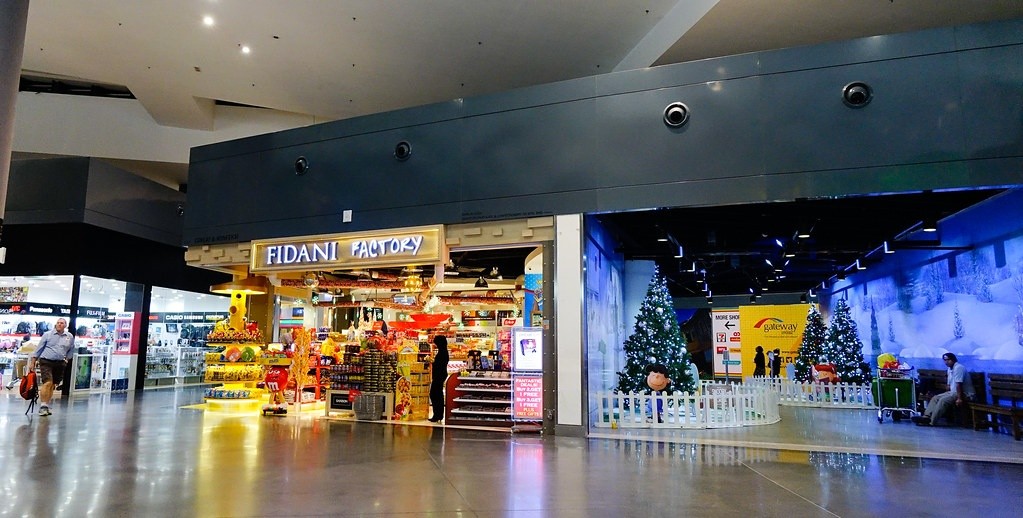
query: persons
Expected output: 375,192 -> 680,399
30,318 -> 74,416
178,329 -> 189,344
911,353 -> 975,426
753,346 -> 765,385
426,335 -> 450,422
767,349 -> 780,386
646,364 -> 670,423
282,327 -> 294,351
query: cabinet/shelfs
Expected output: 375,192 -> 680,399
302,353 -> 329,401
203,341 -> 265,405
111,311 -> 142,354
399,351 -> 432,419
443,372 -> 544,429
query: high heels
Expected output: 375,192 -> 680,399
428,416 -> 443,423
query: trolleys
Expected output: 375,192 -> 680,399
871,365 -> 920,424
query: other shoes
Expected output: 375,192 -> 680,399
916,422 -> 933,426
911,416 -> 931,424
39,406 -> 52,416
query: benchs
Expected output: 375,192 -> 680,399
916,369 -> 988,431
967,373 -> 1023,442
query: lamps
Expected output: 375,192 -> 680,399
357,268 -> 373,282
401,272 -> 421,279
444,259 -> 459,275
401,265 -> 423,272
654,190 -> 938,304
475,274 -> 488,288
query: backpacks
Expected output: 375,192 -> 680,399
20,368 -> 39,400
